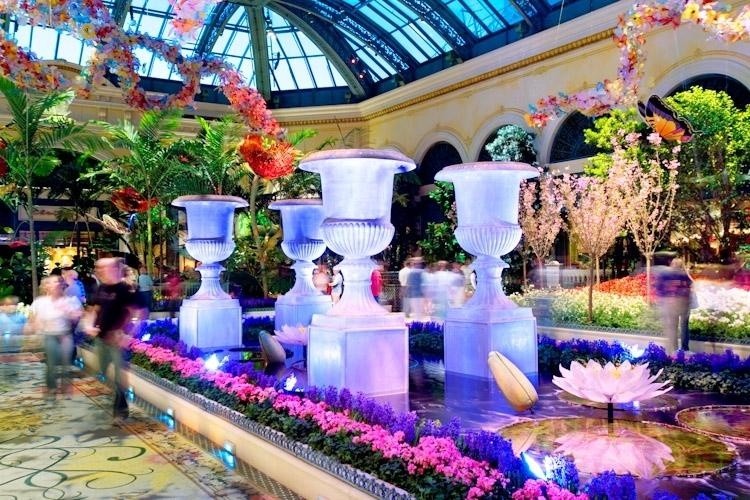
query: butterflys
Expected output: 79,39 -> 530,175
108,184 -> 158,215
84,211 -> 132,236
634,94 -> 704,146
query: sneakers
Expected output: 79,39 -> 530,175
112,416 -> 125,426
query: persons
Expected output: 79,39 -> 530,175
312,258 -> 477,318
1,255 -> 203,428
655,259 -> 696,353
